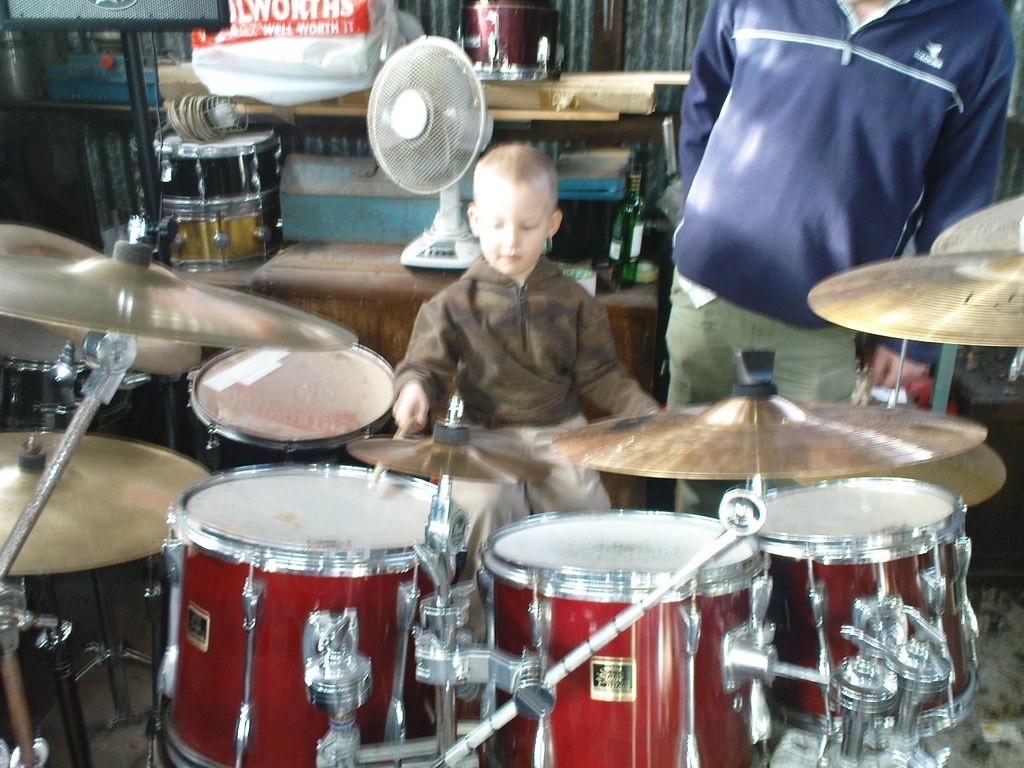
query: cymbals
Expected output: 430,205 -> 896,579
808,249 -> 1024,348
0,430 -> 213,576
346,433 -> 551,484
0,222 -> 203,374
548,393 -> 989,480
791,442 -> 1007,506
929,195 -> 1024,255
0,254 -> 360,354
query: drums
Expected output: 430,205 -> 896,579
753,474 -> 978,746
483,508 -> 761,768
0,317 -> 132,432
153,126 -> 283,199
159,188 -> 281,270
461,0 -> 559,81
189,341 -> 397,474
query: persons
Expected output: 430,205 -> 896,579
665,0 -> 1014,522
393,144 -> 661,703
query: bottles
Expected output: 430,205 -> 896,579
610,172 -> 644,287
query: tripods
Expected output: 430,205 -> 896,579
32,567 -> 153,741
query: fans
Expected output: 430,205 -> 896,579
365,32 -> 494,275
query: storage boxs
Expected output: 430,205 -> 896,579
546,148 -> 634,269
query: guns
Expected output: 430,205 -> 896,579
153,464 -> 468,768
